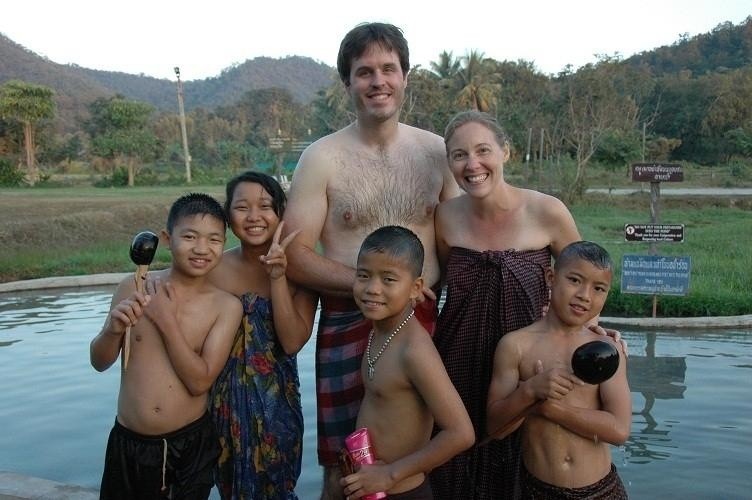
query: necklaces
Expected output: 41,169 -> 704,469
367,309 -> 415,380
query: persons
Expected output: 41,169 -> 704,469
90,195 -> 243,500
486,241 -> 632,500
206,172 -> 320,500
430,110 -> 628,499
340,226 -> 476,500
281,23 -> 461,500
